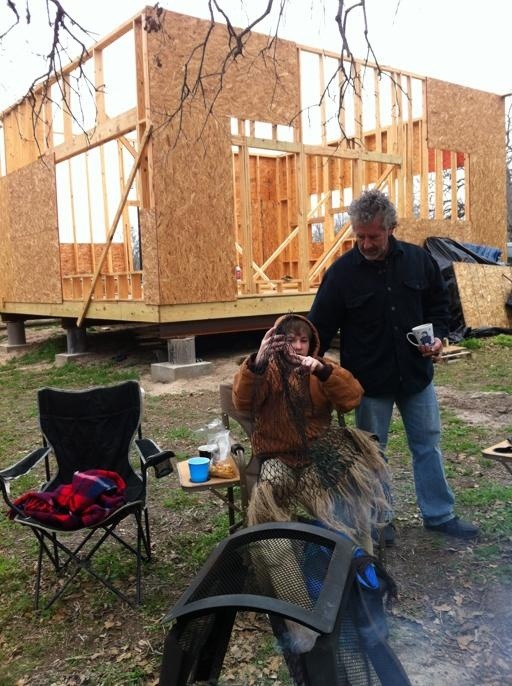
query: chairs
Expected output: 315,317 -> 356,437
0,380 -> 176,614
176,382 -> 385,599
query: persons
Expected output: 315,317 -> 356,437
308,190 -> 481,548
229,310 -> 391,626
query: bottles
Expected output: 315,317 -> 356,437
236,265 -> 241,282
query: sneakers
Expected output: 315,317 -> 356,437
370,521 -> 398,547
423,515 -> 481,539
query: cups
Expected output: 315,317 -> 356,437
188,444 -> 219,483
406,323 -> 435,347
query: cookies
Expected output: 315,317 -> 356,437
211,463 -> 237,479
